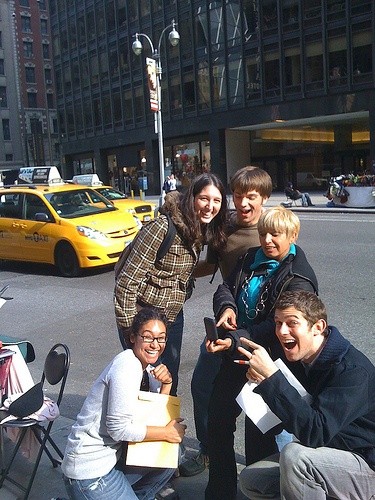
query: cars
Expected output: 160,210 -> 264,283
0,167 -> 162,277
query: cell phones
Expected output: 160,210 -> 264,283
204,317 -> 218,344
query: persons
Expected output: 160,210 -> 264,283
114,156 -> 375,500
51,309 -> 186,500
62,195 -> 86,213
147,64 -> 157,91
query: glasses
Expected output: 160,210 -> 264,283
137,333 -> 168,343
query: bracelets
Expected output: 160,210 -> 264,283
161,380 -> 173,385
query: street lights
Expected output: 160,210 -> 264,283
131,20 -> 181,217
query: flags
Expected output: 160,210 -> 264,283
146,58 -> 159,111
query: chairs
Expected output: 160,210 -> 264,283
285,187 -> 303,207
0,342 -> 71,500
0,199 -> 22,218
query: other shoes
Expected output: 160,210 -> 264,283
175,458 -> 204,477
193,452 -> 209,469
